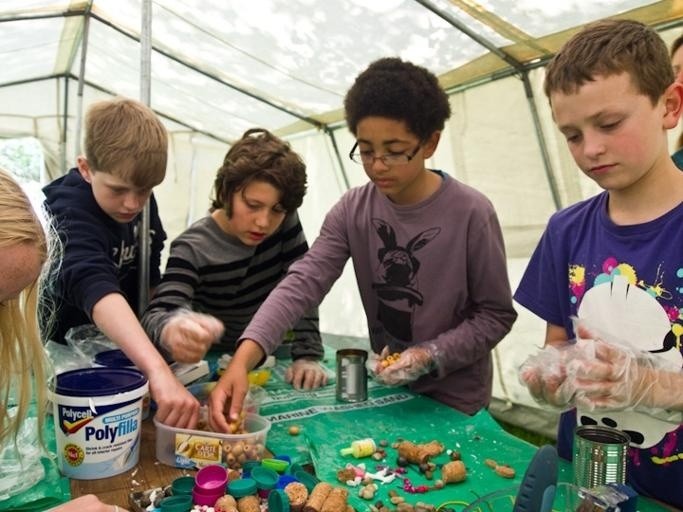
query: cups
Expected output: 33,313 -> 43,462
574,426 -> 631,499
335,347 -> 368,404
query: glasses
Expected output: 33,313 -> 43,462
350,140 -> 423,165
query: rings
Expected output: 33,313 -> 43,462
114,505 -> 119,512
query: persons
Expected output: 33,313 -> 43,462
139,124 -> 330,391
0,171 -> 130,512
513,21 -> 683,511
205,60 -> 517,436
664,27 -> 683,175
32,97 -> 201,431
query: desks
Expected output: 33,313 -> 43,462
0,343 -> 682,511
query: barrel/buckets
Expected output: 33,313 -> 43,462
45,367 -> 150,479
